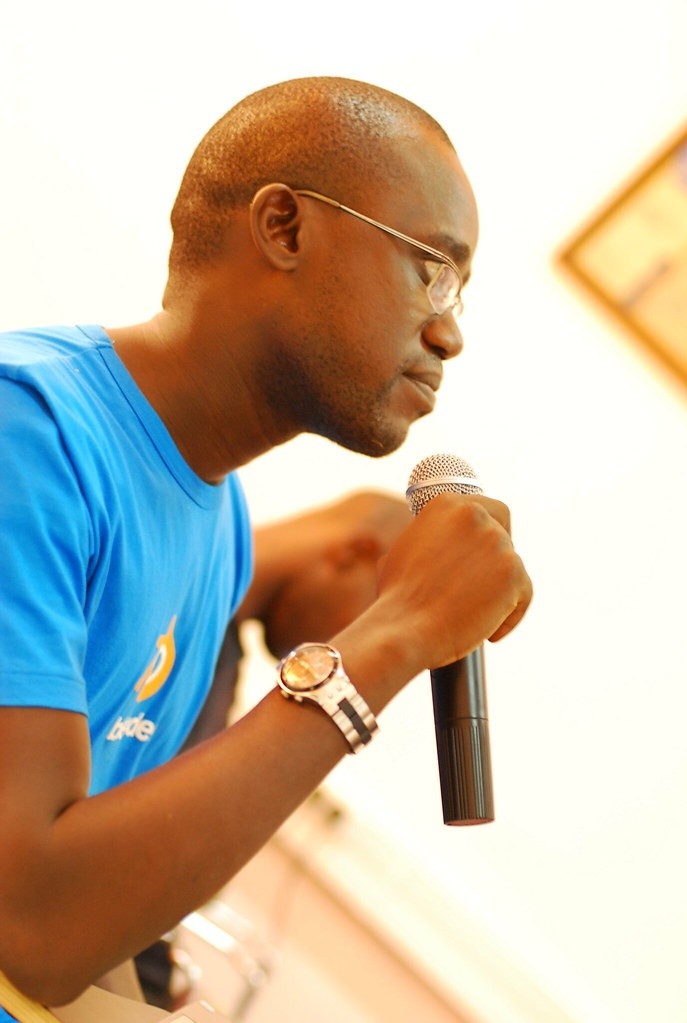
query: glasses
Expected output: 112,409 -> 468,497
282,188 -> 464,327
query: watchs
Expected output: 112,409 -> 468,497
273,640 -> 381,754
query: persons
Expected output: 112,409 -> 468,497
2,77 -> 535,1022
173,492 -> 422,766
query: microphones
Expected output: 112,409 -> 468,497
408,453 -> 496,823
